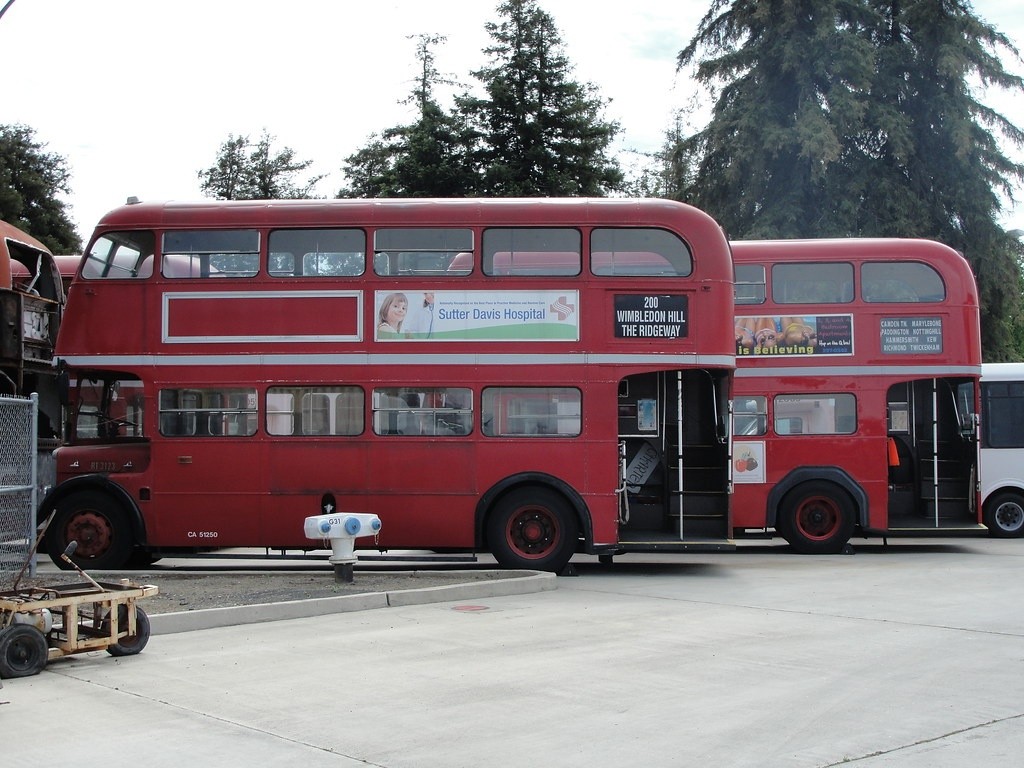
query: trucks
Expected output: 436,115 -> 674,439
733,363 -> 1024,539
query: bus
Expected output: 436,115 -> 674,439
11,253 -> 248,441
420,238 -> 990,552
35,195 -> 737,574
